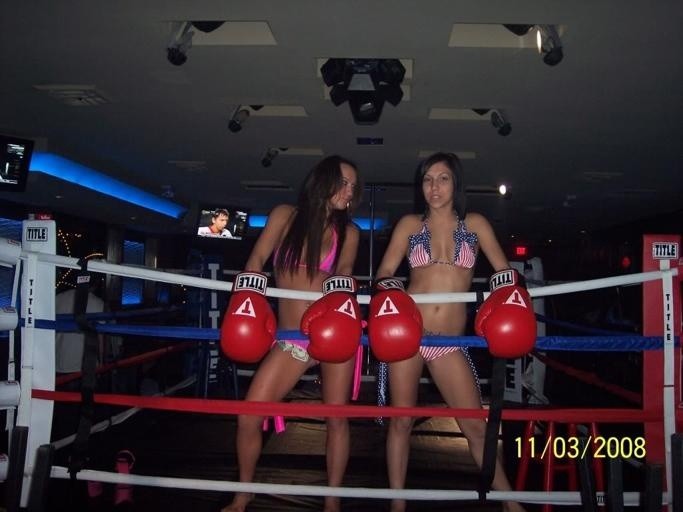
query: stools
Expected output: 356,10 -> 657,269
197,340 -> 239,401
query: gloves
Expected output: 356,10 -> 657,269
475,268 -> 538,361
365,276 -> 423,361
300,274 -> 362,363
218,271 -> 277,366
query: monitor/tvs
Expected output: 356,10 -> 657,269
0,133 -> 36,193
193,205 -> 250,243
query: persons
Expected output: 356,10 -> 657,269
220,155 -> 362,512
197,208 -> 232,238
367,151 -> 537,512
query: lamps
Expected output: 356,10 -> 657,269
227,104 -> 277,167
166,21 -> 195,65
488,110 -> 512,137
535,24 -> 563,66
